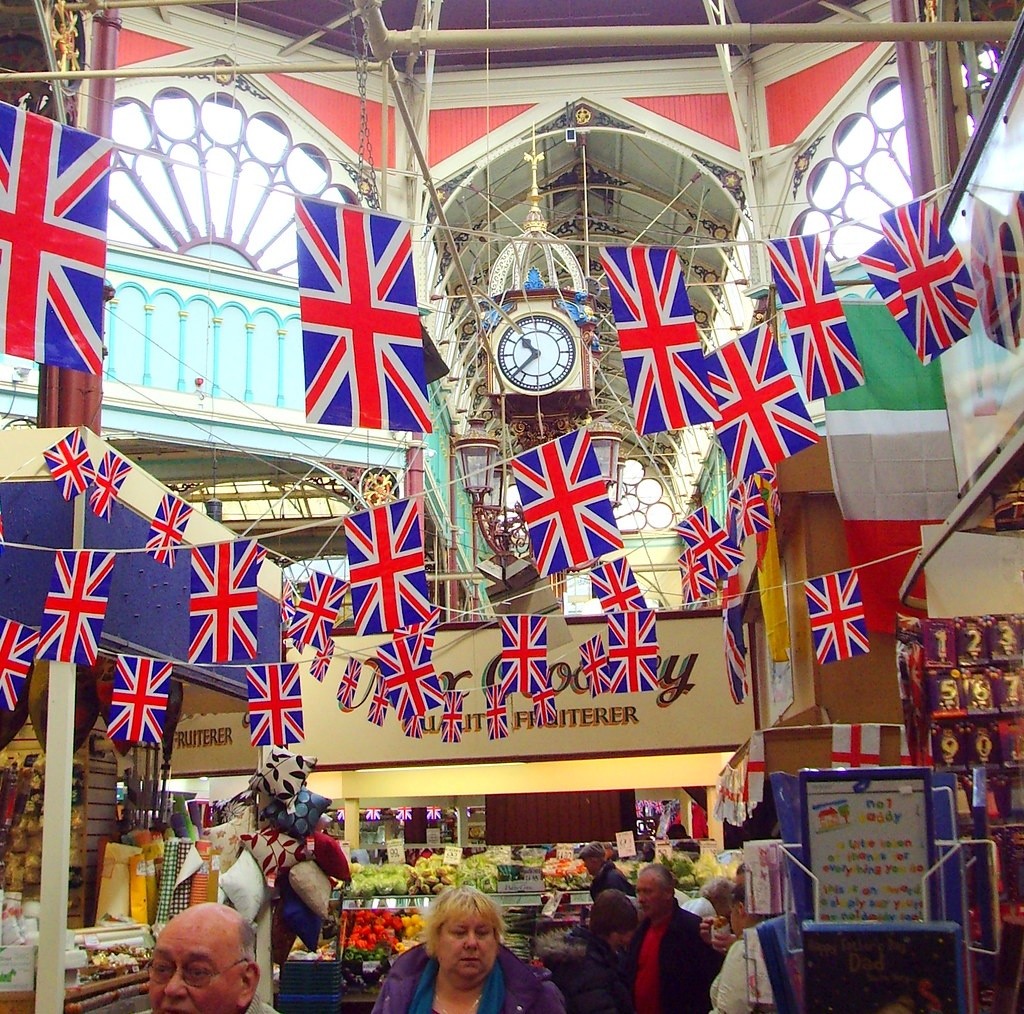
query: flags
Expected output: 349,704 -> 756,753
0,617 -> 41,711
858,237 -> 957,366
366,467 -> 937,827
704,322 -> 821,478
146,494 -> 192,570
972,192 -> 1024,356
0,499 -> 6,557
294,194 -> 432,433
279,571 -> 360,710
764,234 -> 866,402
0,101 -> 114,376
108,655 -> 171,746
34,550 -> 115,665
44,429 -> 132,524
188,537 -> 257,661
879,201 -> 972,354
925,198 -> 979,322
599,244 -> 723,435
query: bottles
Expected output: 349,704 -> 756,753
1,908 -> 24,944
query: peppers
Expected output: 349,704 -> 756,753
345,910 -> 425,961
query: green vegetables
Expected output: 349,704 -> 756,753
628,851 -> 694,892
459,849 -> 544,893
545,871 -> 593,890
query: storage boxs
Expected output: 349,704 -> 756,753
274,958 -> 343,1014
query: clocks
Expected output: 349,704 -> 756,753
495,312 -> 579,395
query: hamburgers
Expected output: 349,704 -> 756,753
714,915 -> 727,931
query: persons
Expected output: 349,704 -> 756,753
137,902 -> 283,1014
369,824 -> 766,1014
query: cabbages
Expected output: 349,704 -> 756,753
349,864 -> 408,897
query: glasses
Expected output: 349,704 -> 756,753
148,958 -> 249,987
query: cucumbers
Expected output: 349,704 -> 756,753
502,913 -> 535,962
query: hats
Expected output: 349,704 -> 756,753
577,841 -> 605,859
